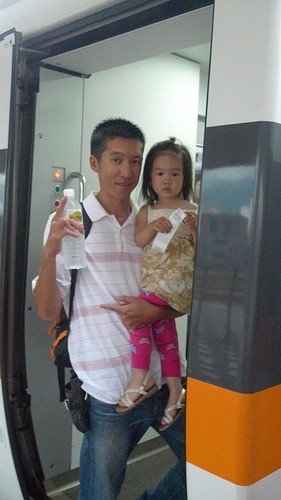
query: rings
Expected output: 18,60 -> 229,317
137,311 -> 142,319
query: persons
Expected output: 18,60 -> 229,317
116,138 -> 199,431
32,118 -> 187,500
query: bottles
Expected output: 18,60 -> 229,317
60,189 -> 88,269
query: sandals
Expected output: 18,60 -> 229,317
116,370 -> 158,413
159,388 -> 186,431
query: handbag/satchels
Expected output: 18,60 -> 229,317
49,319 -> 89,433
